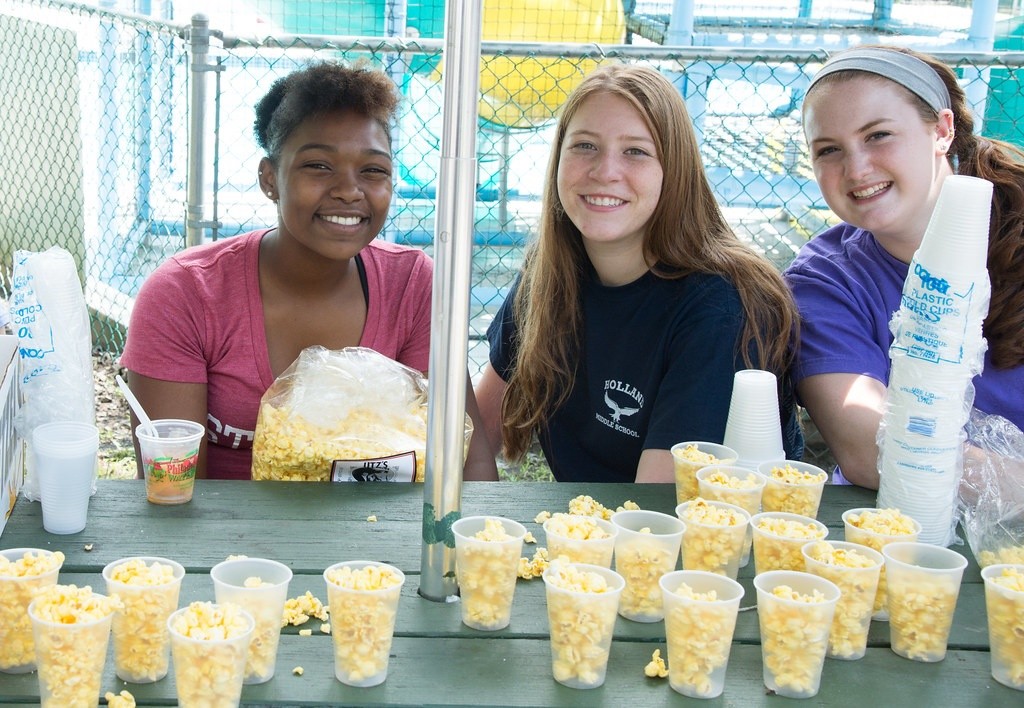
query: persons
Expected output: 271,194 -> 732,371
474,63 -> 805,483
120,59 -> 499,480
780,44 -> 1024,491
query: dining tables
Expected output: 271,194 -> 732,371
0,481 -> 1024,708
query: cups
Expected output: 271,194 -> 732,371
0,175 -> 1024,707
